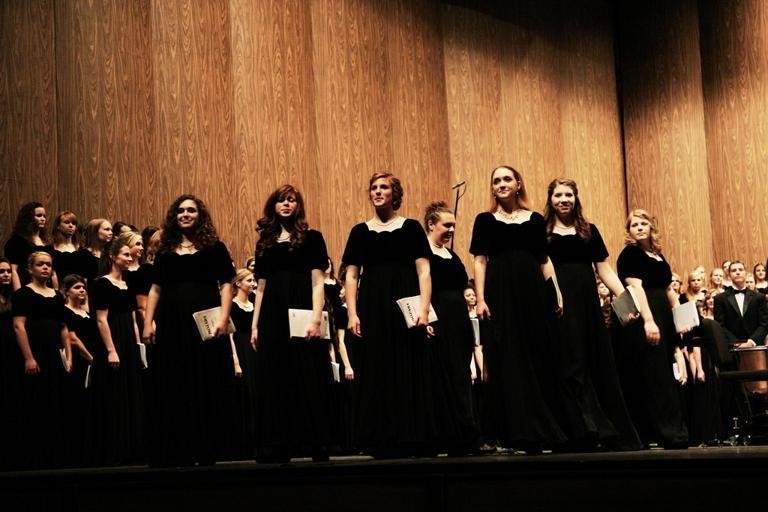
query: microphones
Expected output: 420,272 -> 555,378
452,180 -> 466,190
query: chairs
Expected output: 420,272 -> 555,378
700,318 -> 768,446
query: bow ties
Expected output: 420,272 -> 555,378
734,289 -> 747,294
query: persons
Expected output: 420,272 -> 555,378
0,165 -> 768,466
